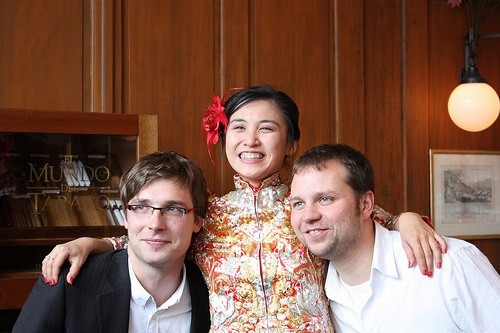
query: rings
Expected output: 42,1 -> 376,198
44,255 -> 55,260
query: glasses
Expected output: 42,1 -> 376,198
125,203 -> 197,219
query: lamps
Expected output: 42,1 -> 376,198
447,0 -> 500,133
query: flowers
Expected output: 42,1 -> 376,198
200,86 -> 245,166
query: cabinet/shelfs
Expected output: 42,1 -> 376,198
0,106 -> 160,309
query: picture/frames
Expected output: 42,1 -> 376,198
428,147 -> 500,242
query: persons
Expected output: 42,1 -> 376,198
42,85 -> 447,333
12,151 -> 211,333
290,143 -> 500,333
